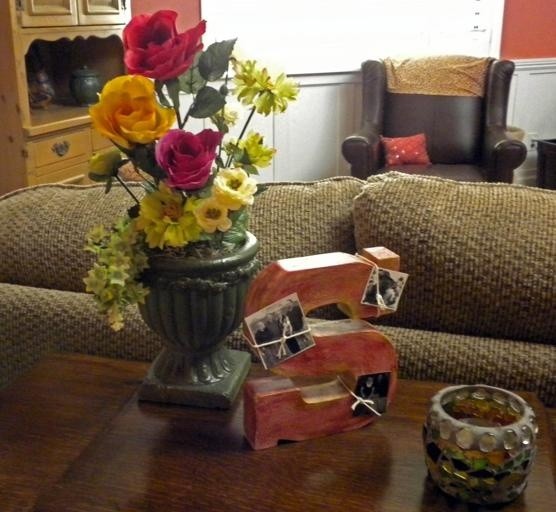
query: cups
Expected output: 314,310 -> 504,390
424,383 -> 540,504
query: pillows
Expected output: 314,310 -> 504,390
379,131 -> 432,168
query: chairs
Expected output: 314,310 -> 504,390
340,56 -> 528,185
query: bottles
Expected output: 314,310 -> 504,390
27,70 -> 57,109
69,64 -> 103,107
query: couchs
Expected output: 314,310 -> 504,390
1,170 -> 556,406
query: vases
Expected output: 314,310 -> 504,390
124,242 -> 263,409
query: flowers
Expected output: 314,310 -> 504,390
81,8 -> 299,332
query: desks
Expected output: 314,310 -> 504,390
1,352 -> 556,510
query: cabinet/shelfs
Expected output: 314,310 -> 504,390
1,0 -> 135,188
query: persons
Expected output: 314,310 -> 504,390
366,271 -> 403,306
264,314 -> 284,360
353,376 -> 377,415
255,321 -> 277,365
274,311 -> 301,354
286,299 -> 307,349
